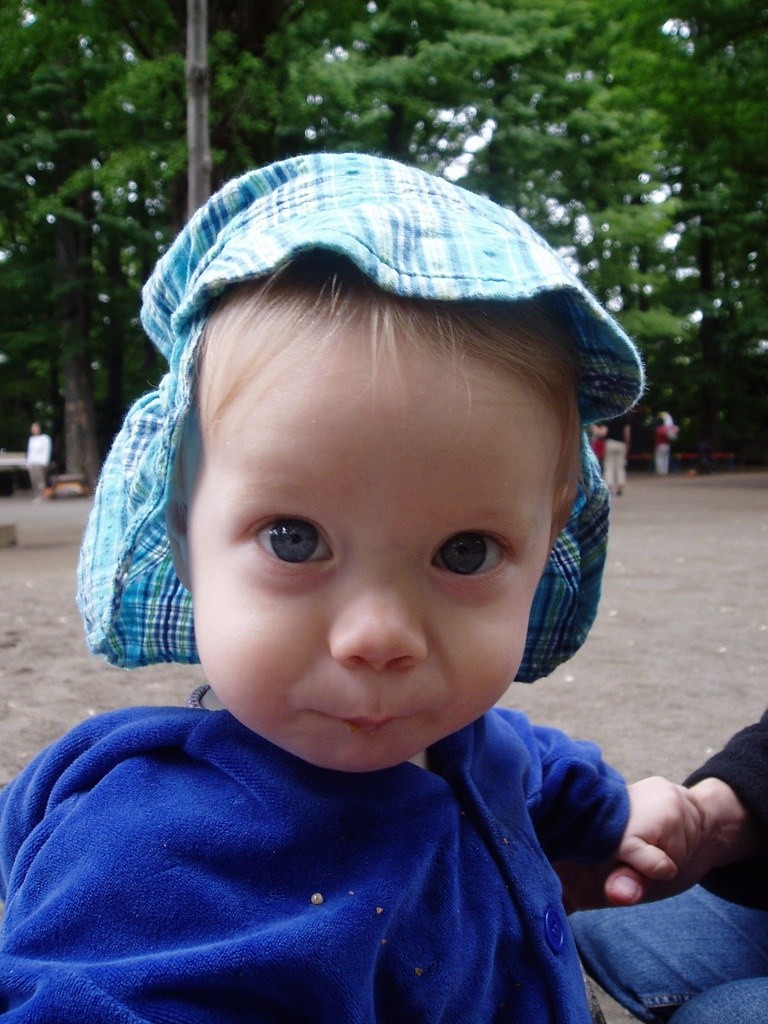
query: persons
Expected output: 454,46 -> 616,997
25,420 -> 52,500
567,707 -> 768,1024
0,152 -> 706,1024
591,406 -> 675,495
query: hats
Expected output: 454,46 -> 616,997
72,152 -> 646,684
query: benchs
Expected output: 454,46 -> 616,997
674,453 -> 735,472
628,452 -> 653,472
